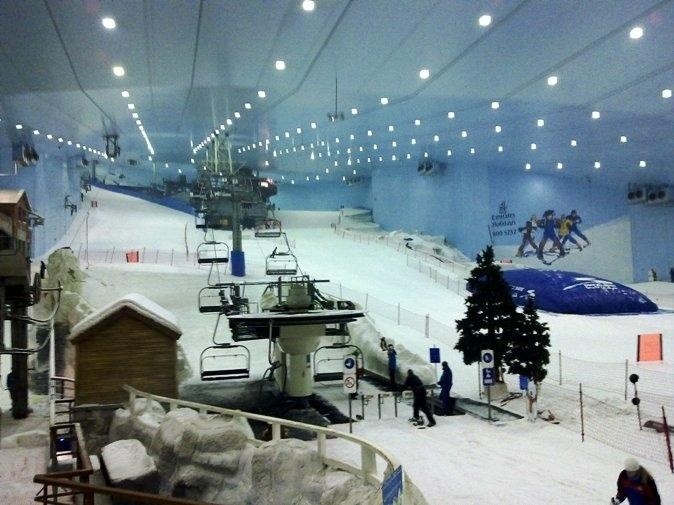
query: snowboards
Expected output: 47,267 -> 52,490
409,416 -> 424,430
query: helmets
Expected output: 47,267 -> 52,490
624,458 -> 639,472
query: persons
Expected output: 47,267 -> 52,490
387,344 -> 397,389
516,209 -> 589,259
609,456 -> 661,505
393,369 -> 436,427
437,361 -> 453,416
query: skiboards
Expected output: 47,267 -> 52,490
515,242 -> 590,265
